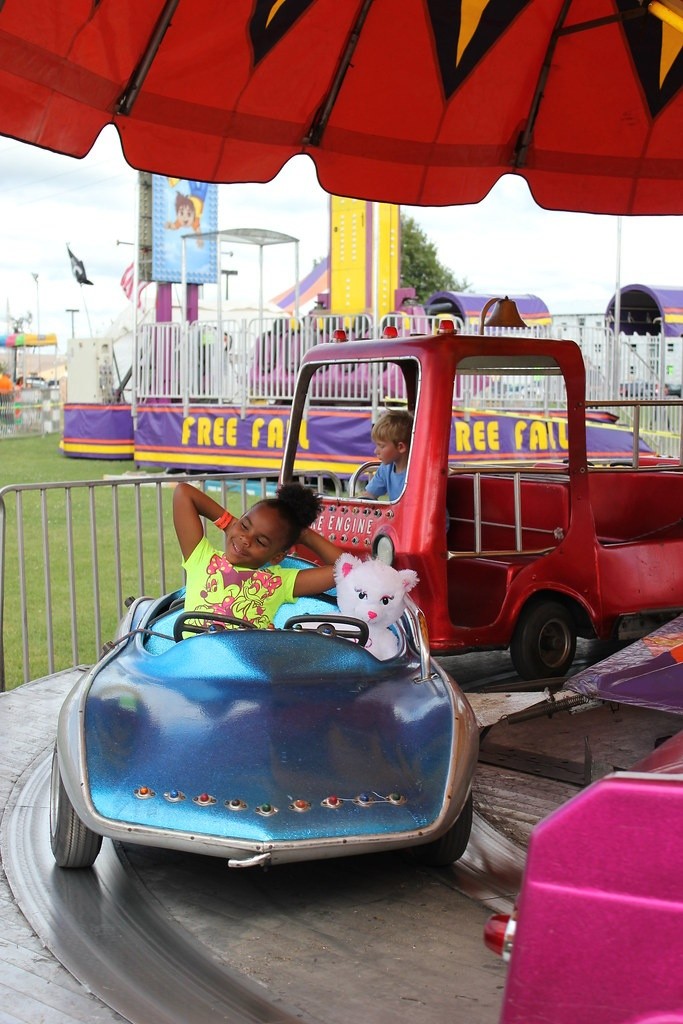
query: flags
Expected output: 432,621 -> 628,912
68,248 -> 93,285
121,263 -> 152,307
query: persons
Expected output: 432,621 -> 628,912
15,377 -> 30,389
0,372 -> 14,403
361,411 -> 449,536
173,481 -> 344,640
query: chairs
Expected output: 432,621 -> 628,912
145,556 -> 400,657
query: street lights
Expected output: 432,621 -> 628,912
222,269 -> 239,301
64,309 -> 80,338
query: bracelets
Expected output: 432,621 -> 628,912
212,511 -> 234,530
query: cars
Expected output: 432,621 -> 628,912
47,557 -> 482,871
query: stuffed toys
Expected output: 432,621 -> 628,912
299,553 -> 420,659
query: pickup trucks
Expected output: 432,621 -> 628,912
270,314 -> 682,680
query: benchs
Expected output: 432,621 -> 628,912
346,460 -> 683,628
